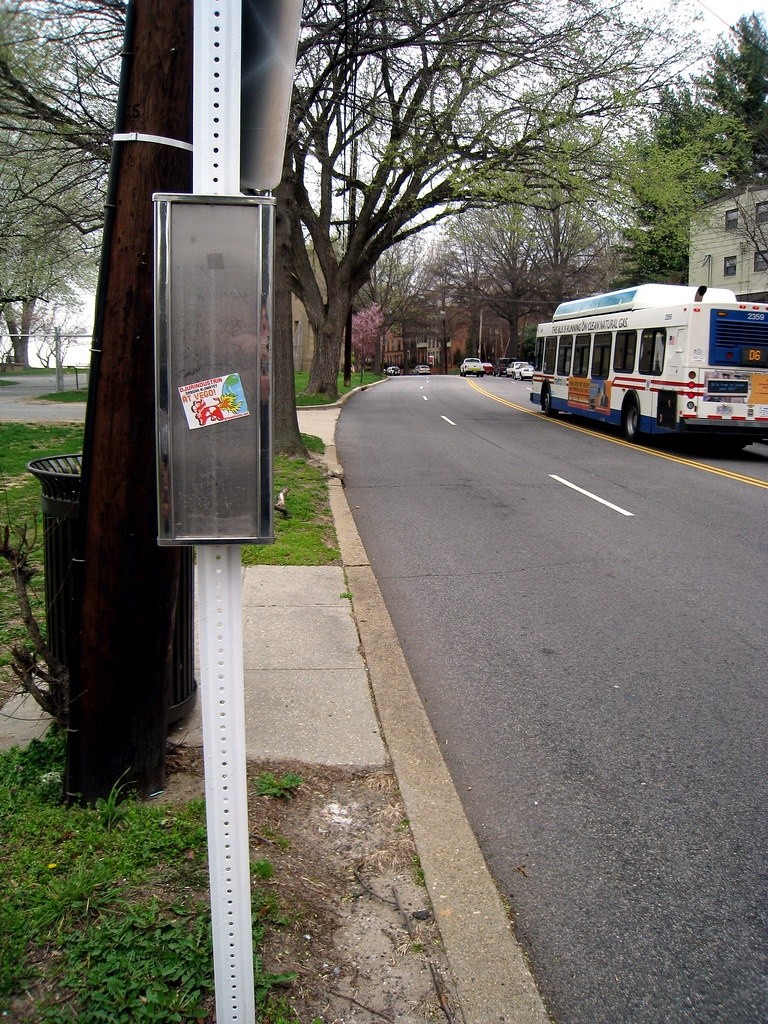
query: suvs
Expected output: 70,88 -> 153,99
492,358 -> 517,377
506,362 -> 529,379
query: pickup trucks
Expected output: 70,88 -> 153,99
458,358 -> 485,378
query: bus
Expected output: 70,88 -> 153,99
526,283 -> 768,443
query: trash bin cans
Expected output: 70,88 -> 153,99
25,452 -> 197,724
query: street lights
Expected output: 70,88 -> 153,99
440,309 -> 448,375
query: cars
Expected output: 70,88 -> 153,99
383,367 -> 401,375
483,363 -> 493,375
412,365 -> 432,375
514,365 -> 534,380
